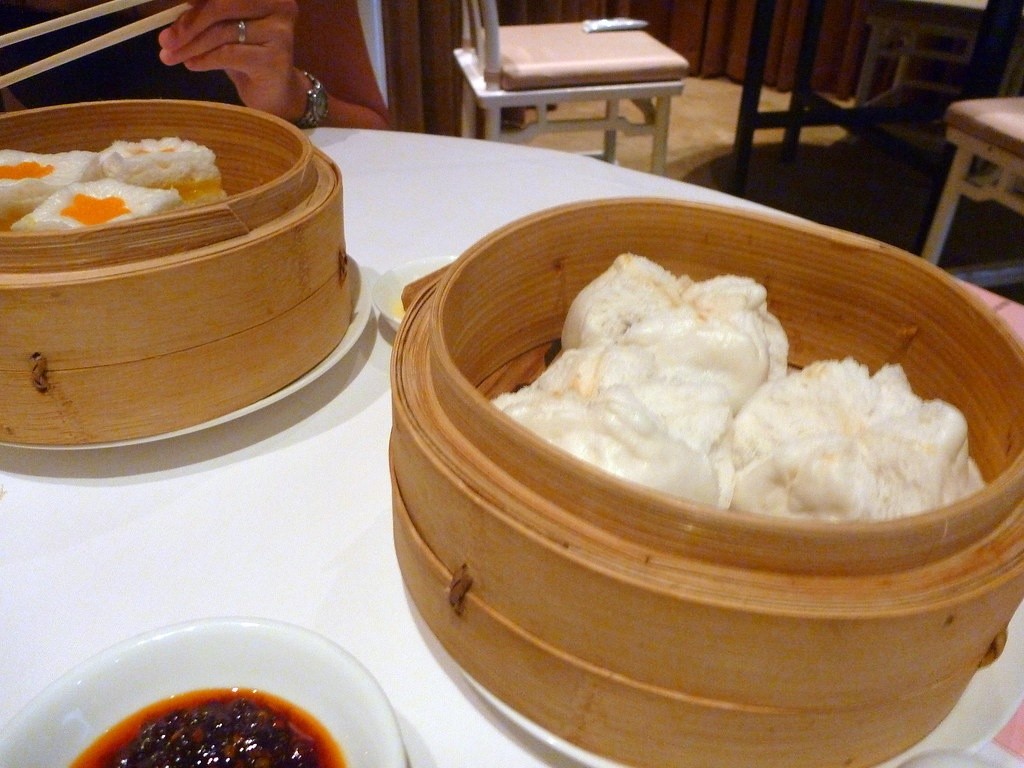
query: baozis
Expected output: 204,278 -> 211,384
485,251 -> 984,522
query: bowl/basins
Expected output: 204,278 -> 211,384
0,616 -> 407,768
371,255 -> 458,332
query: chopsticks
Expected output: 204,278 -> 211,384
0,0 -> 194,86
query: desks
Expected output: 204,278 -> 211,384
724,0 -> 1024,257
0,128 -> 1024,768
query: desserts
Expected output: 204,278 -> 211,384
0,135 -> 228,232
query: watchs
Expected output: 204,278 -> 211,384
297,73 -> 328,129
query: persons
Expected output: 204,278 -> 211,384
0,0 -> 397,129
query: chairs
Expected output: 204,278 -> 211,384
921,96 -> 1024,288
453,0 -> 690,177
847,0 -> 1024,149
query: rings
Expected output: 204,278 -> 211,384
238,20 -> 245,44
